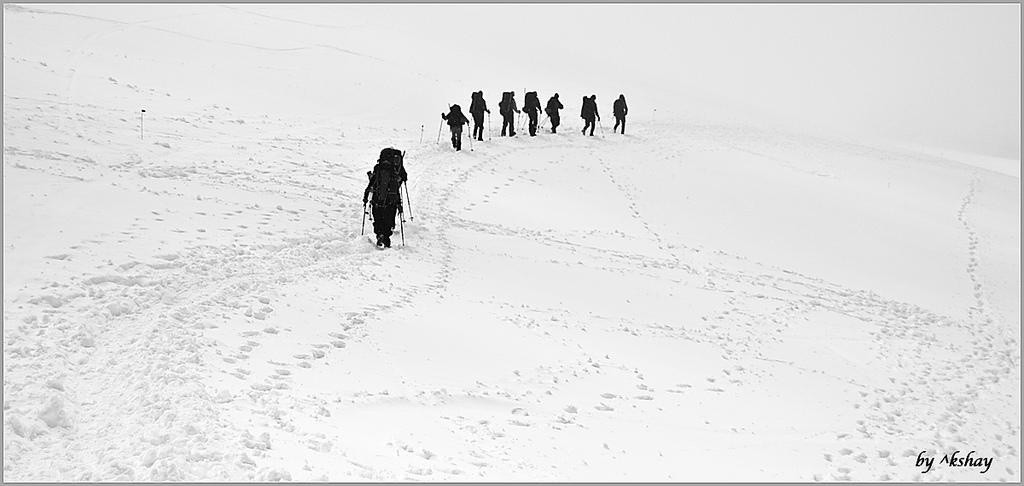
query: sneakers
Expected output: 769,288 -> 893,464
473,134 -> 483,141
453,142 -> 461,151
551,127 -> 556,133
376,234 -> 391,249
614,127 -> 624,134
529,128 -> 537,136
501,131 -> 517,137
582,130 -> 594,136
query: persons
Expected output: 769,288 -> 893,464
612,93 -> 628,134
498,90 -> 521,137
580,93 -> 601,136
470,88 -> 490,141
525,90 -> 543,136
441,104 -> 470,152
360,156 -> 403,249
373,159 -> 407,236
549,92 -> 564,133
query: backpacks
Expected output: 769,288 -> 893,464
372,148 -> 404,212
544,97 -> 558,117
500,92 -> 513,116
469,92 -> 482,114
447,104 -> 461,126
582,96 -> 595,119
614,99 -> 625,117
522,92 -> 537,113
